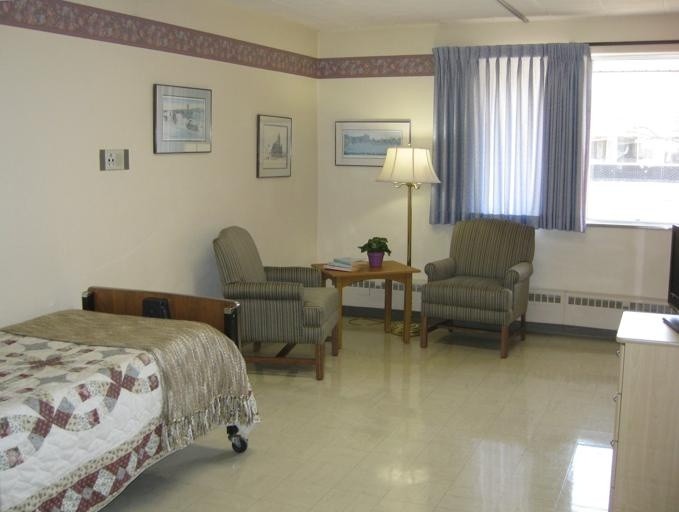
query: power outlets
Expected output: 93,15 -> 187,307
101,148 -> 118,172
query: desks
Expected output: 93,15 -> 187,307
313,260 -> 418,346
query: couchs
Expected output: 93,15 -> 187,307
420,217 -> 536,359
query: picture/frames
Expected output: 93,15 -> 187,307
153,83 -> 215,156
335,122 -> 412,169
258,113 -> 295,179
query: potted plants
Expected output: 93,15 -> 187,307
359,232 -> 393,267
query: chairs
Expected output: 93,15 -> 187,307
213,227 -> 345,380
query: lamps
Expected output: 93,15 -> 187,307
377,146 -> 442,343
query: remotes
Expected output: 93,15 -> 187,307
324,265 -> 350,272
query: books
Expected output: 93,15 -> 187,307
323,256 -> 369,273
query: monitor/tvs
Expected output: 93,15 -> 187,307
663,225 -> 679,333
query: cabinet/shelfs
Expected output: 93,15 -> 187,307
608,310 -> 679,511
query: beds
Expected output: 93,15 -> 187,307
0,287 -> 258,511
